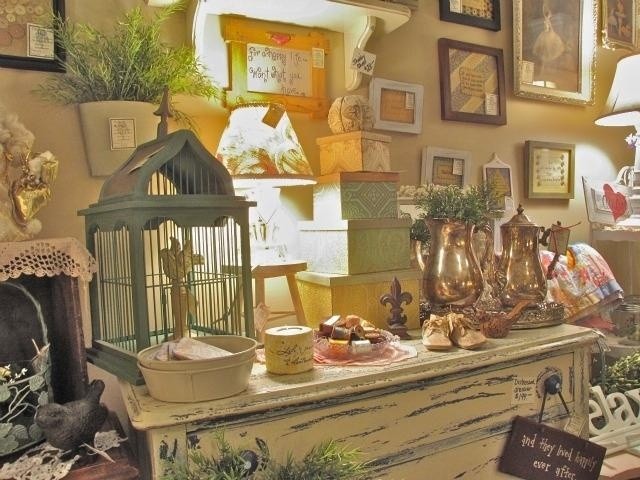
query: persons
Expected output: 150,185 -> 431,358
532,0 -> 565,87
608,0 -> 632,42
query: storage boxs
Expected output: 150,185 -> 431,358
290,130 -> 430,333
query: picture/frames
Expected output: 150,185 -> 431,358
437,37 -> 509,127
0,0 -> 69,74
420,144 -> 474,193
482,152 -> 514,215
368,76 -> 424,135
599,0 -> 640,56
219,24 -> 331,121
511,1 -> 600,107
524,140 -> 578,201
438,0 -> 502,33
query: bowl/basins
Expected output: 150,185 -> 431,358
135,333 -> 258,404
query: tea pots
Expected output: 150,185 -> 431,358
408,215 -> 486,311
492,205 -> 562,307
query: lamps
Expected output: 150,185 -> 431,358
213,102 -> 320,265
594,55 -> 639,201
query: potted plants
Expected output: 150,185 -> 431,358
28,1 -> 225,179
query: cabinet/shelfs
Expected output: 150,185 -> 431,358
114,322 -> 604,479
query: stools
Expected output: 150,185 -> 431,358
218,259 -> 309,343
